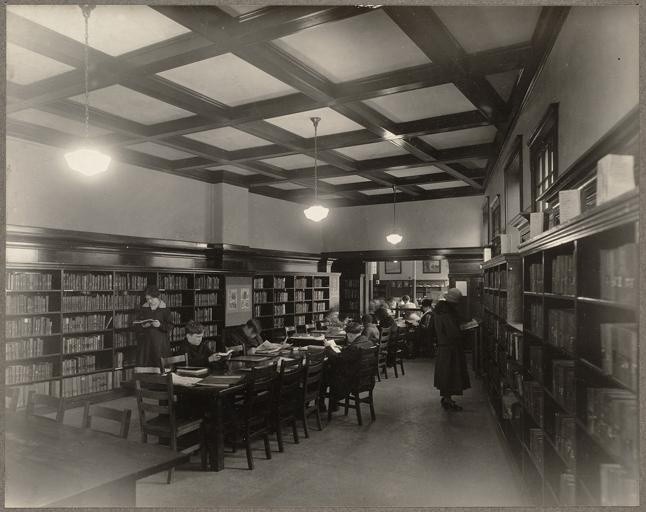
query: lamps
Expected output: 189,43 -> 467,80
62,4 -> 112,176
304,116 -> 330,224
385,185 -> 403,244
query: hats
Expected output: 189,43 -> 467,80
343,323 -> 361,333
441,288 -> 465,304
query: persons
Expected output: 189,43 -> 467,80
225,319 -> 263,355
316,288 -> 477,411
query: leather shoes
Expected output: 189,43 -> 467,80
440,397 -> 463,411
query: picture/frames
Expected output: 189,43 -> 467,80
422,260 -> 441,273
384,260 -> 402,274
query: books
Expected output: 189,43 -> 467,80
549,256 -> 576,507
592,245 -> 646,509
4,269 -> 220,411
478,263 -> 542,465
253,277 -> 326,333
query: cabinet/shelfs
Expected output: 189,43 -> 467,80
342,278 -> 364,321
3,264 -> 223,409
252,271 -> 341,333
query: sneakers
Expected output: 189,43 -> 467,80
317,402 -> 340,412
